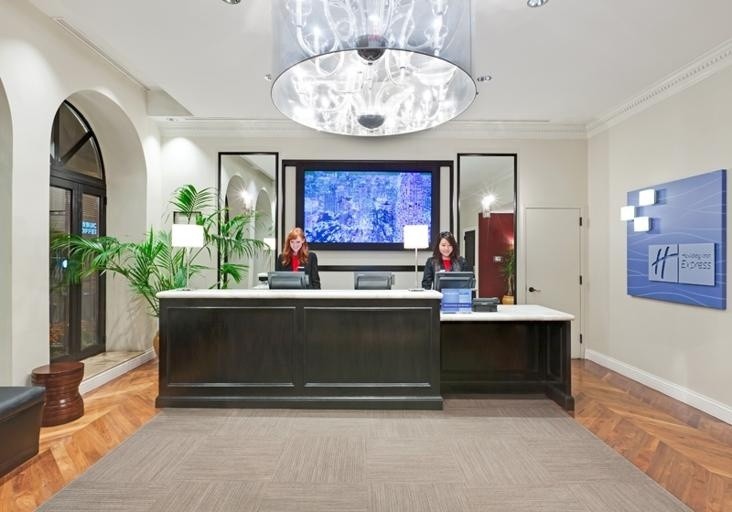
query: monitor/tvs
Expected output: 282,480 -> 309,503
353,270 -> 396,290
267,271 -> 310,289
434,271 -> 476,291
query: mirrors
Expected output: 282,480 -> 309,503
217,151 -> 278,291
457,152 -> 517,307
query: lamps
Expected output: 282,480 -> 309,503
172,223 -> 204,291
262,236 -> 275,271
267,0 -> 479,136
403,224 -> 429,288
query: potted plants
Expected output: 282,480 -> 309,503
49,182 -> 266,361
498,252 -> 516,306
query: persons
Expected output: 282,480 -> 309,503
422,230 -> 474,289
276,226 -> 321,289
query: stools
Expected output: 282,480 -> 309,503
31,362 -> 84,427
0,386 -> 46,480
